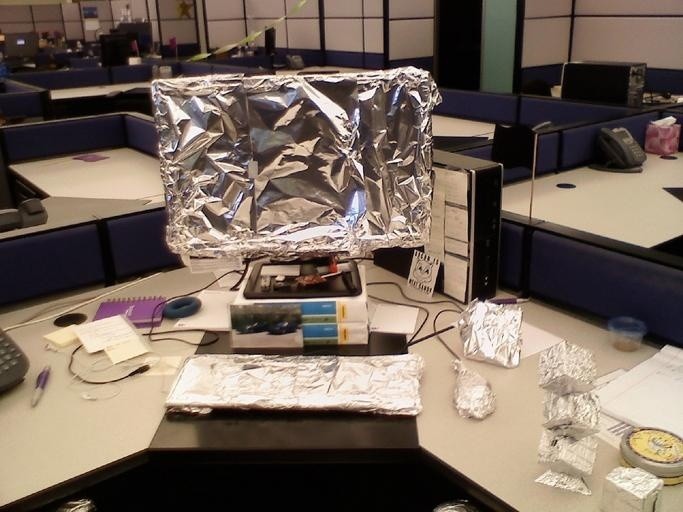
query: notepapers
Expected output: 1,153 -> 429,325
44,322 -> 79,348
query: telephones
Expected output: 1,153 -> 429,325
589,126 -> 647,173
285,54 -> 305,69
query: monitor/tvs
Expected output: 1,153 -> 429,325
115,22 -> 154,57
5,32 -> 40,66
100,34 -> 139,67
151,65 -> 443,298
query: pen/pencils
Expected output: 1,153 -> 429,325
32,365 -> 50,406
489,298 -> 530,304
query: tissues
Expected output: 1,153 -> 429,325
645,115 -> 681,155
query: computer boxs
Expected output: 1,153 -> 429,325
559,60 -> 647,107
374,149 -> 505,304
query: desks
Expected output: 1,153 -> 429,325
2,109 -> 188,312
419,92 -> 683,349
7,62 -> 261,102
0,263 -> 677,511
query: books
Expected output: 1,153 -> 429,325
93,296 -> 165,328
599,344 -> 682,440
